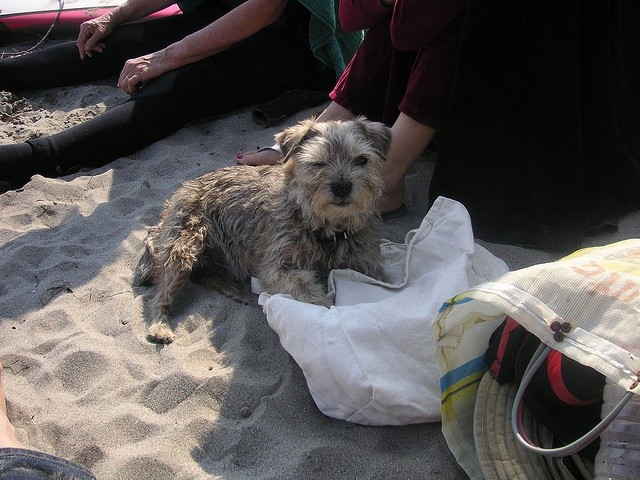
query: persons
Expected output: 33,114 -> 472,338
235,0 -> 467,215
0,0 -> 370,195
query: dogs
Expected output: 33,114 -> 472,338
131,115 -> 393,345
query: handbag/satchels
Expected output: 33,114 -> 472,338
430,237 -> 639,474
249,197 -> 510,427
